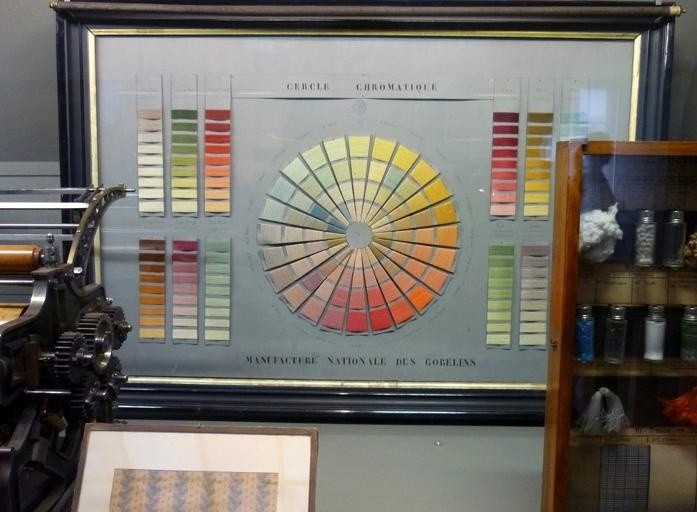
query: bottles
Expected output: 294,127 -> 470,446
574,304 -> 596,364
680,305 -> 696,362
602,304 -> 628,366
643,305 -> 666,360
664,210 -> 688,268
633,209 -> 657,268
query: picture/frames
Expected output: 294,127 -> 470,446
68,420 -> 317,512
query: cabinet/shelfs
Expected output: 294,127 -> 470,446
539,137 -> 697,512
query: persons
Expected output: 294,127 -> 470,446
579,131 -> 637,237
